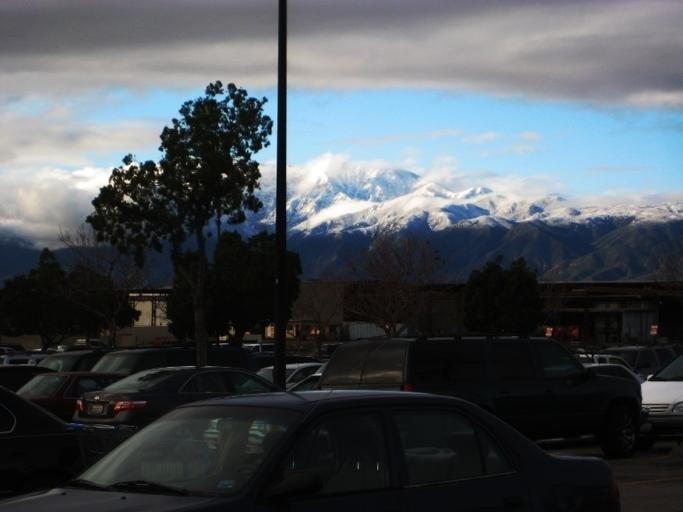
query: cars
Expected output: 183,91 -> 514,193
0,387 -> 622,512
0,336 -> 683,498
